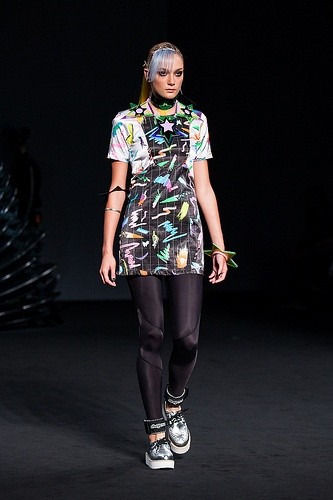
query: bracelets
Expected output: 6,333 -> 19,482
104,208 -> 122,215
204,243 -> 238,268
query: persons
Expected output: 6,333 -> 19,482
99,41 -> 238,470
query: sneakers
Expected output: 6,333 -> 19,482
161,400 -> 191,455
145,438 -> 175,470
